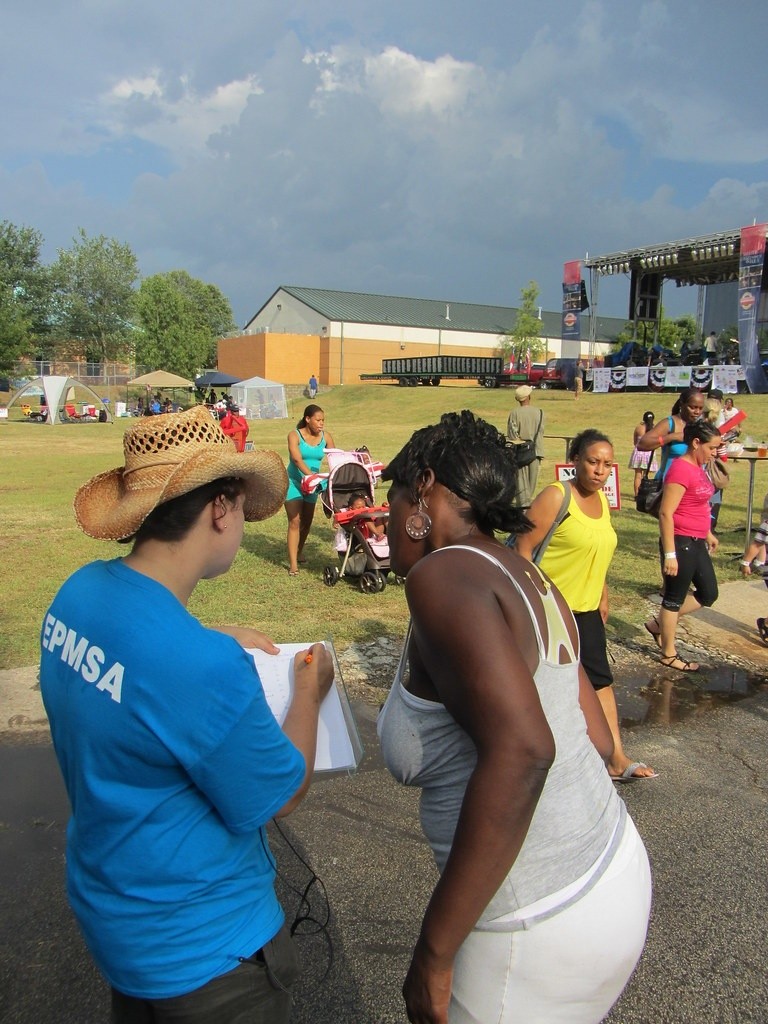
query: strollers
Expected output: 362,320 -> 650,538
318,451 -> 406,594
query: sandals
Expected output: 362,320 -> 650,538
644,616 -> 661,648
757,617 -> 768,645
658,651 -> 698,671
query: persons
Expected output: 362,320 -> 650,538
380,412 -> 654,1023
37,401 -> 47,421
638,388 -> 743,669
137,397 -> 172,417
740,519 -> 768,644
508,385 -> 545,513
309,375 -> 317,399
195,388 -> 228,404
355,446 -> 372,464
574,360 -> 589,400
629,412 -> 659,496
515,429 -> 660,780
283,404 -> 335,577
256,390 -> 265,404
348,493 -> 389,541
704,331 -> 728,365
41,408 -> 334,1024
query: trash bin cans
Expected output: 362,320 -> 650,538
0,379 -> 9,392
40,395 -> 44,405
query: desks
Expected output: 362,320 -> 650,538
542,435 -> 574,464
714,455 -> 768,561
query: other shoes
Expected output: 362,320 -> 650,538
713,531 -> 722,536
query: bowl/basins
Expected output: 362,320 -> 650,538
743,446 -> 759,452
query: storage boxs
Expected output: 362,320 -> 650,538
114,402 -> 132,417
88,405 -> 95,416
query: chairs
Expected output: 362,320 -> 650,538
249,404 -> 261,418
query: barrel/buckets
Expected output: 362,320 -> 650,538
40,394 -> 45,404
65,404 -> 76,416
114,402 -> 126,417
83,405 -> 107,422
20,404 -> 31,414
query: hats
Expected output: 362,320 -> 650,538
515,385 -> 532,401
708,389 -> 723,400
72,406 -> 290,541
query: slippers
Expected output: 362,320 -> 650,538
610,761 -> 659,780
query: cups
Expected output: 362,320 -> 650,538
758,443 -> 767,458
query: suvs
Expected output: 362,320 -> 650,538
478,360 -> 552,389
543,357 -> 603,389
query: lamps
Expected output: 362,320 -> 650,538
597,242 -> 739,287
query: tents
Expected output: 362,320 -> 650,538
6,376 -> 115,423
195,372 -> 244,394
127,370 -> 194,411
231,376 -> 288,419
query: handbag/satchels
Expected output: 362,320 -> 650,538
510,438 -> 536,468
706,457 -> 730,489
636,477 -> 663,519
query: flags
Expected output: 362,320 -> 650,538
510,345 -> 530,379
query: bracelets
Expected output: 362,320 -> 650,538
665,553 -> 676,558
658,436 -> 664,446
741,560 -> 749,566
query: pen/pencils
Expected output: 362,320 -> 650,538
305,655 -> 312,664
